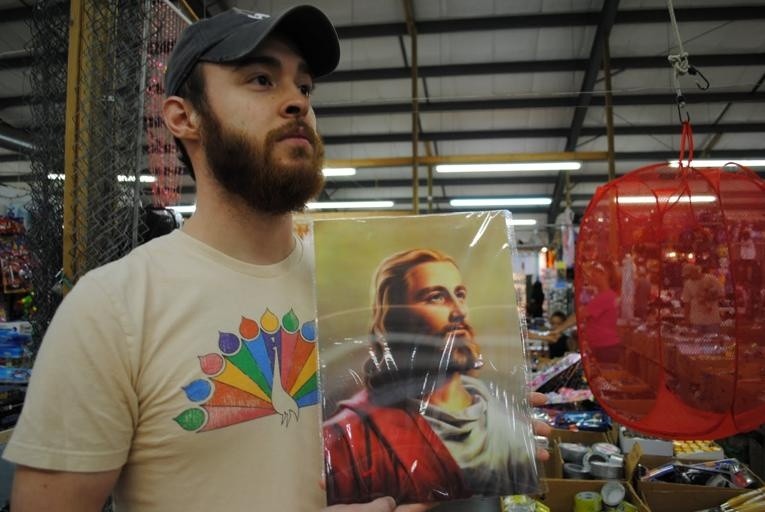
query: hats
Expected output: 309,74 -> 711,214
163,4 -> 341,99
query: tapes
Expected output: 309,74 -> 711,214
557,442 -> 638,512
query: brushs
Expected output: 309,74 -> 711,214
688,485 -> 765,512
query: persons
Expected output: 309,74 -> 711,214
634,217 -> 757,291
546,310 -> 572,358
682,262 -> 727,334
0,1 -> 556,510
547,257 -> 622,367
319,248 -> 544,502
632,265 -> 653,320
